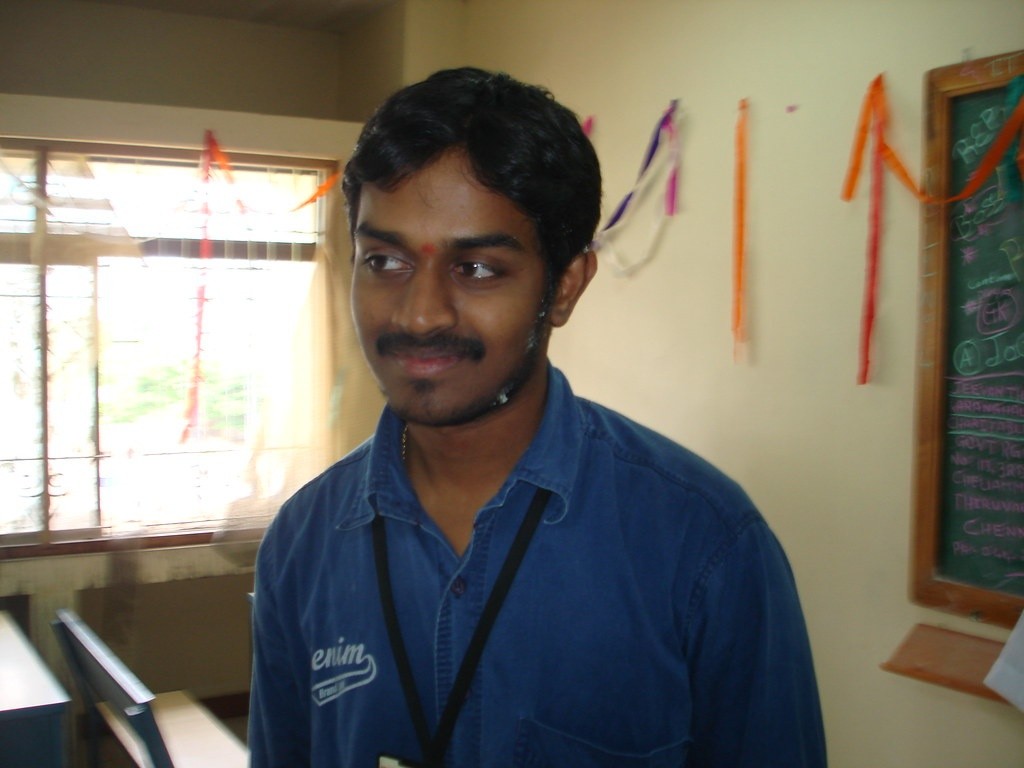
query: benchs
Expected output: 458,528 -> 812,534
50,608 -> 251,768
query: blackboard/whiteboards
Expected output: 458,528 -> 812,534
905,42 -> 1024,634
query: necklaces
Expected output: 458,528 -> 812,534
399,422 -> 410,463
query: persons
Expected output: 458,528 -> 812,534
246,65 -> 831,768
